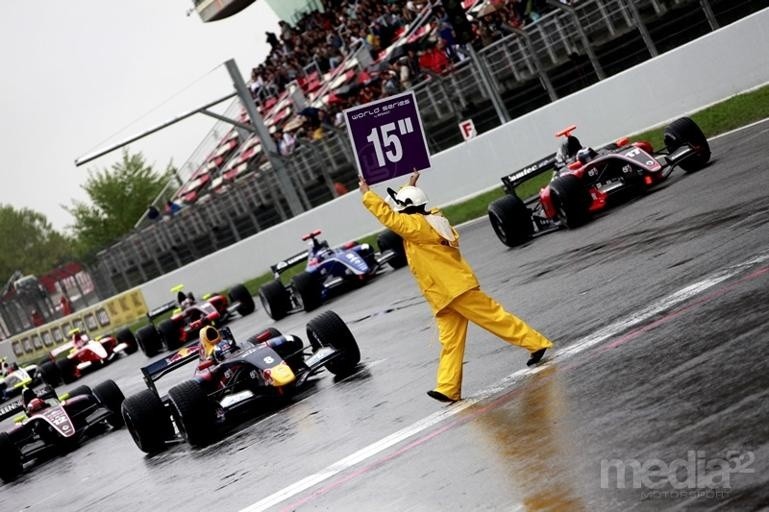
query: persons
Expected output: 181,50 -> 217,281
31,308 -> 44,328
250,0 -> 581,158
357,167 -> 555,402
167,198 -> 181,214
59,296 -> 75,316
551,134 -> 587,181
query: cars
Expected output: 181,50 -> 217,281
0,225 -> 409,486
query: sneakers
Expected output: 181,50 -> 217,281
427,391 -> 454,402
527,347 -> 546,366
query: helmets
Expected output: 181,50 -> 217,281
382,186 -> 428,212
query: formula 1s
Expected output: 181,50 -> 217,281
0,225 -> 409,486
